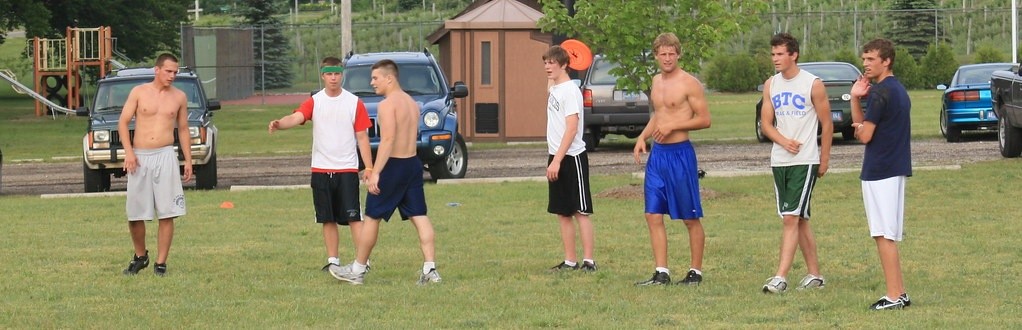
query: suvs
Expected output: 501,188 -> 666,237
581,50 -> 661,152
75,65 -> 221,194
309,46 -> 470,183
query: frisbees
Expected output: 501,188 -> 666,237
560,39 -> 592,71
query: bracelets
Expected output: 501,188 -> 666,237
365,167 -> 372,171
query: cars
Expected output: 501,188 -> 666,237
936,62 -> 1022,143
990,62 -> 1022,158
754,61 -> 873,143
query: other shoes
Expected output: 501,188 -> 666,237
322,263 -> 340,271
366,265 -> 370,273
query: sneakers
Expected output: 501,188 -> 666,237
677,270 -> 702,285
762,276 -> 789,293
551,262 -> 579,272
329,264 -> 365,285
123,250 -> 150,275
869,293 -> 910,310
636,271 -> 671,286
796,274 -> 826,291
154,262 -> 167,277
416,268 -> 442,287
580,261 -> 597,273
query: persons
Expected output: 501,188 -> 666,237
760,32 -> 833,294
328,60 -> 442,286
542,45 -> 599,275
268,57 -> 373,275
850,40 -> 911,312
117,53 -> 193,280
630,33 -> 711,288
